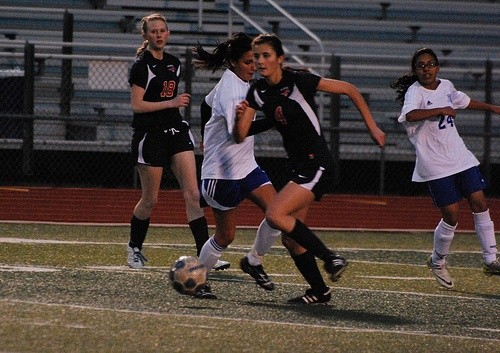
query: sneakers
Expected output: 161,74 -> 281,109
196,256 -> 230,271
427,255 -> 454,289
195,284 -> 218,300
239,256 -> 274,290
483,261 -> 500,276
127,240 -> 147,269
286,286 -> 332,305
324,253 -> 348,281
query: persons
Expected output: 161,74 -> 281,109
233,34 -> 385,306
398,48 -> 500,289
127,13 -> 230,270
189,34 -> 280,292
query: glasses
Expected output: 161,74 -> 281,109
415,60 -> 436,70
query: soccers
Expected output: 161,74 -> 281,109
169,255 -> 207,297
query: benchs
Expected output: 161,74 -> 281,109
0,1 -> 500,163
